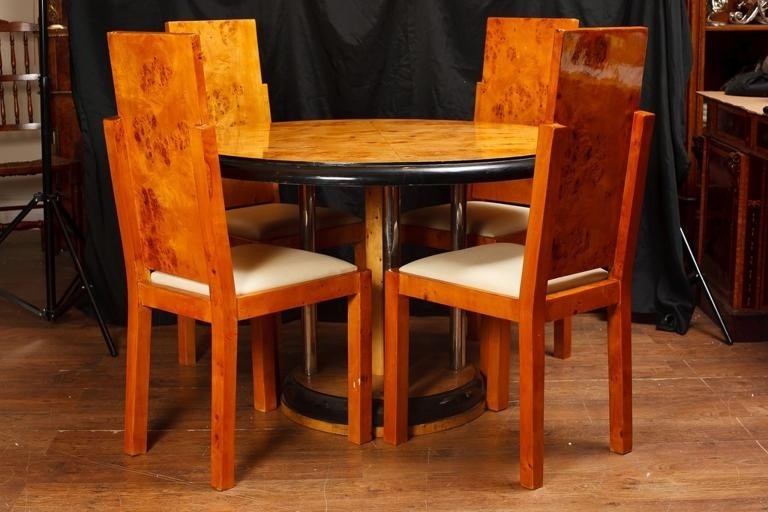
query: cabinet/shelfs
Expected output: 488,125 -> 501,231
678,0 -> 768,277
698,92 -> 768,342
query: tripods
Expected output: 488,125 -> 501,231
0,0 -> 121,359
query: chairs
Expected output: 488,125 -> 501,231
0,20 -> 47,251
163,19 -> 361,412
103,32 -> 372,491
384,27 -> 655,490
398,16 -> 580,374
